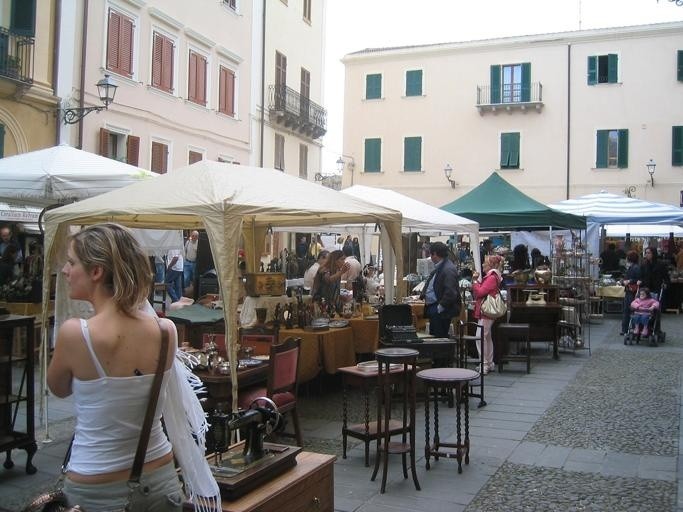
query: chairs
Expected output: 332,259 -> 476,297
238,336 -> 302,448
378,303 -> 413,340
238,326 -> 280,357
148,255 -> 168,313
201,325 -> 225,351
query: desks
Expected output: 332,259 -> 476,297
331,314 -> 419,355
506,285 -> 563,361
1,300 -> 55,363
258,322 -> 355,400
407,302 -> 466,333
370,347 -> 422,494
594,285 -> 625,299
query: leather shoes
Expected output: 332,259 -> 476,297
620,330 -> 627,335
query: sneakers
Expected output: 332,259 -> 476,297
632,325 -> 638,334
485,364 -> 495,372
475,365 -> 490,375
641,327 -> 649,335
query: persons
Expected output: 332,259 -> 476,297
23,242 -> 43,279
340,246 -> 361,286
45,224 -> 185,512
599,244 -> 618,273
154,255 -> 164,285
0,229 -> 23,286
620,251 -> 640,336
334,236 -> 362,257
183,231 -> 200,296
419,242 -> 460,365
312,250 -> 350,309
631,289 -> 660,336
473,256 -> 504,376
456,269 -> 472,302
295,236 -> 308,259
456,239 -> 494,259
273,245 -> 298,273
531,249 -> 549,273
164,249 -> 183,304
637,247 -> 667,299
303,250 -> 328,293
309,236 -> 322,259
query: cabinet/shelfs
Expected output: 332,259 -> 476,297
184,452 -> 339,511
0,315 -> 39,475
547,236 -> 594,356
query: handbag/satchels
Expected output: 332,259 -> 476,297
480,274 -> 507,319
19,316 -> 168,511
629,284 -> 638,292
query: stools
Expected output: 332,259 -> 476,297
337,365 -> 422,468
496,323 -> 530,375
416,367 -> 480,474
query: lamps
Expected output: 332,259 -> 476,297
443,164 -> 457,188
55,72 -> 120,125
646,159 -> 656,187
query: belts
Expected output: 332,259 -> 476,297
185,257 -> 197,264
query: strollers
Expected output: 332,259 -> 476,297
624,284 -> 666,347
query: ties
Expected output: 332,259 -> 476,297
419,267 -> 436,298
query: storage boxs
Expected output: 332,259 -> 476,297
246,272 -> 285,296
